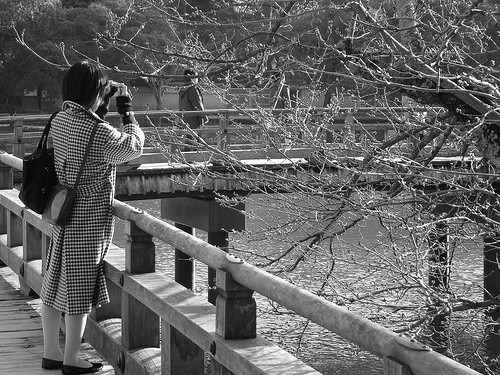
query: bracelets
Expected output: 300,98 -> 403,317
121,111 -> 134,118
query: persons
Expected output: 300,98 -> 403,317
179,70 -> 209,150
41,60 -> 145,375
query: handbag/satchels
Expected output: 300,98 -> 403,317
42,184 -> 76,226
18,148 -> 58,214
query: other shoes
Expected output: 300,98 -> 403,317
61,361 -> 104,375
41,357 -> 63,371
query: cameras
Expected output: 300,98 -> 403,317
105,86 -> 118,98
